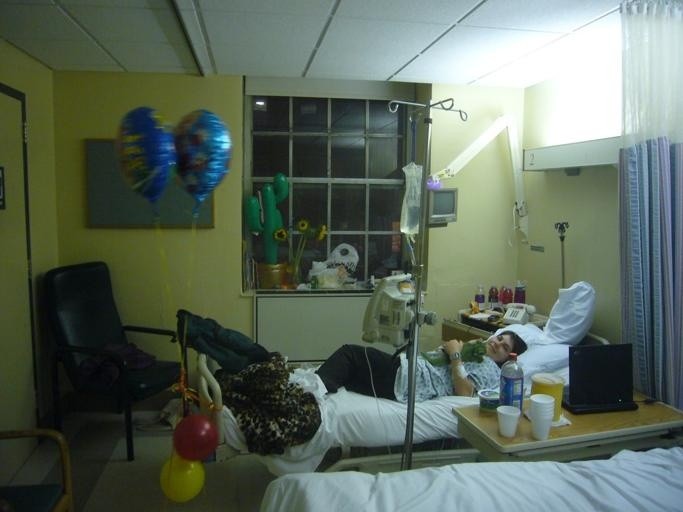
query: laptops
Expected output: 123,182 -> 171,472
561,343 -> 638,415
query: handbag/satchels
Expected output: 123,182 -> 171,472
160,398 -> 198,431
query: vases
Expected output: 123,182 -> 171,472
282,258 -> 303,286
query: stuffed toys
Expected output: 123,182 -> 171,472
421,340 -> 485,367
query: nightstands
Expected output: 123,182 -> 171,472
440,306 -> 549,344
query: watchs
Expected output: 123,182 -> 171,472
449,352 -> 462,361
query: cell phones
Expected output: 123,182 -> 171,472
488,315 -> 500,322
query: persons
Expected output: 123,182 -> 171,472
314,330 -> 528,406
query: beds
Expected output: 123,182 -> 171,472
193,317 -> 617,479
252,432 -> 681,511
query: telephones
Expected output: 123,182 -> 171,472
501,303 -> 536,325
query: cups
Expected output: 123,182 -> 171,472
497,405 -> 521,437
528,393 -> 555,441
530,372 -> 566,420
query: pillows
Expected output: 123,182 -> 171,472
490,321 -> 572,378
538,278 -> 597,344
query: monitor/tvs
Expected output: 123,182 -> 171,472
427,187 -> 458,224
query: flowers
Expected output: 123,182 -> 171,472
275,219 -> 325,284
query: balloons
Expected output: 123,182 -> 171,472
158,413 -> 220,502
115,105 -> 179,219
173,109 -> 232,218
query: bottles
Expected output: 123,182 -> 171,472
498,282 -> 512,305
487,284 -> 498,310
513,279 -> 528,305
474,283 -> 485,312
499,353 -> 524,413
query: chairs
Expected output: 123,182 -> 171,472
0,428 -> 72,512
41,257 -> 189,464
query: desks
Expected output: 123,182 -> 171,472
451,388 -> 681,464
